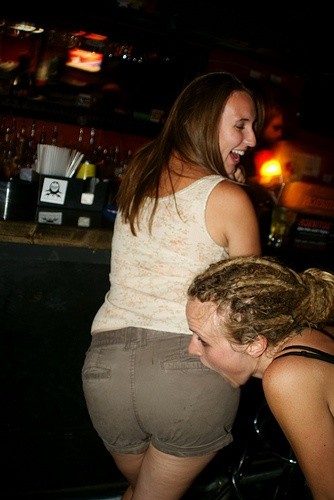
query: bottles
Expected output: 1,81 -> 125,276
0,174 -> 14,219
73,161 -> 97,206
1,119 -> 136,182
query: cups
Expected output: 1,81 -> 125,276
28,169 -> 81,210
267,205 -> 296,243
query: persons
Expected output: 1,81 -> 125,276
80,72 -> 263,499
238,100 -> 283,176
184,253 -> 333,500
9,54 -> 32,86
